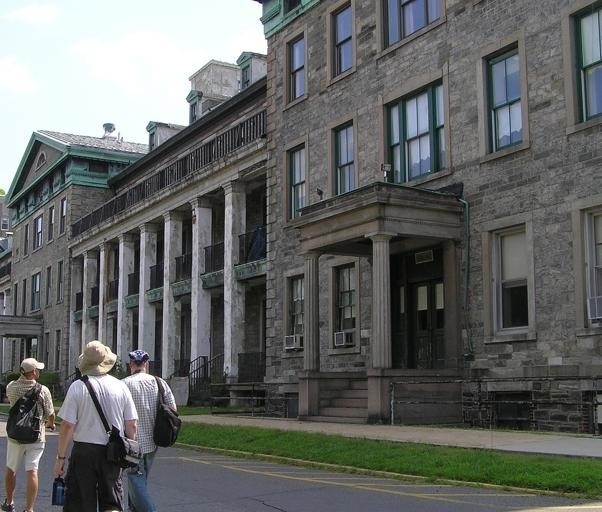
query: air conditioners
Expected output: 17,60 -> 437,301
285,334 -> 303,351
333,331 -> 355,347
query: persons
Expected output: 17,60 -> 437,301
0,358 -> 56,512
55,340 -> 139,512
121,350 -> 178,512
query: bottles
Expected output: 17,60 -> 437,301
51,474 -> 65,505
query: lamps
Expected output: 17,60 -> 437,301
317,187 -> 323,201
381,162 -> 393,182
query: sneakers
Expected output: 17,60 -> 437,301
0,498 -> 15,511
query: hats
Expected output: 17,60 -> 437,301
129,349 -> 150,364
20,358 -> 44,374
76,341 -> 118,374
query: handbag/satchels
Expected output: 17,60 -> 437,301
6,396 -> 42,443
106,425 -> 143,467
153,401 -> 182,447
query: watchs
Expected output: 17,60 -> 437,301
55,454 -> 66,460
